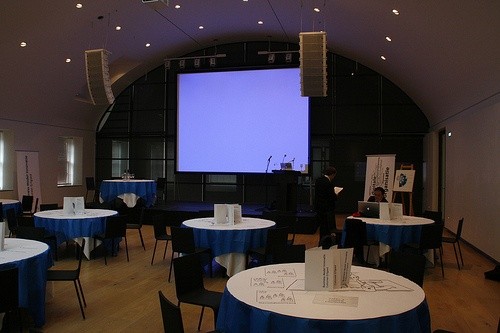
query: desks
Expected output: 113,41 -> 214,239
97,179 -> 158,214
182,217 -> 276,280
215,264 -> 432,333
0,236 -> 52,333
345,214 -> 435,266
33,209 -> 118,265
0,199 -> 23,237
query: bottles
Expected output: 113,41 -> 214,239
123,169 -> 130,181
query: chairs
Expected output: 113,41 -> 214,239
22,194 -> 171,266
422,210 -> 464,277
46,238 -> 86,320
262,197 -> 305,265
151,210 -> 224,333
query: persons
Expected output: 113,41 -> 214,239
367,187 -> 388,202
315,167 -> 339,249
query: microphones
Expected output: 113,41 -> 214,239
280,155 -> 296,170
268,156 -> 272,161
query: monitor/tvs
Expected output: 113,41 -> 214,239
357,202 -> 380,218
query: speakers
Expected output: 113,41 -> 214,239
299,32 -> 327,96
84,48 -> 115,106
